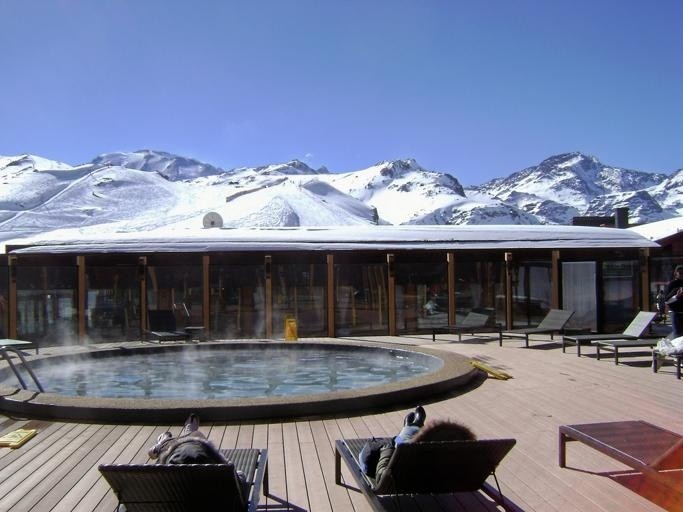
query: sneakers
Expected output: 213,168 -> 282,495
148,432 -> 172,459
185,414 -> 198,433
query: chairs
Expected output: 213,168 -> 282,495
498,308 -> 575,348
97,445 -> 271,511
588,337 -> 668,366
333,437 -> 521,512
431,306 -> 503,345
142,307 -> 191,344
560,309 -> 660,358
555,419 -> 683,495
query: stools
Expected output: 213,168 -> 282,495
648,347 -> 683,380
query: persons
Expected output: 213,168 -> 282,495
657,336 -> 682,356
665,265 -> 682,354
147,412 -> 245,485
375,405 -> 477,487
656,289 -> 665,320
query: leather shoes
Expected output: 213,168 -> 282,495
404,405 -> 426,426
